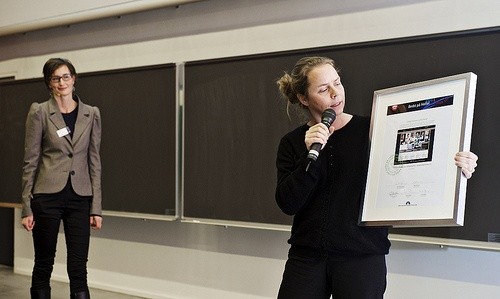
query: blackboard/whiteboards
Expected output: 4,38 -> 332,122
181,26 -> 500,250
0,63 -> 178,221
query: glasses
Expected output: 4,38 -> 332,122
50,73 -> 74,82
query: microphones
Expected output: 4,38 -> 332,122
304,108 -> 336,173
51,88 -> 61,97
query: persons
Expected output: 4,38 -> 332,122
274,56 -> 478,299
20,58 -> 103,299
388,105 -> 402,115
